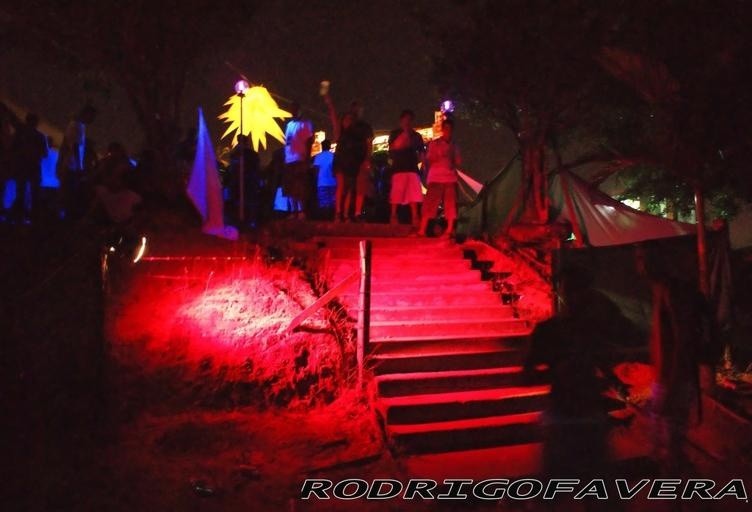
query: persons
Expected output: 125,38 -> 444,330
518,245 -> 706,512
229,93 -> 462,239
8,111 -> 98,218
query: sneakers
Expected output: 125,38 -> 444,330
362,210 -> 365,215
335,214 -> 352,225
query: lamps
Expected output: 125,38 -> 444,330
235,79 -> 249,223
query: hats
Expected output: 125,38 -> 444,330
442,100 -> 454,113
